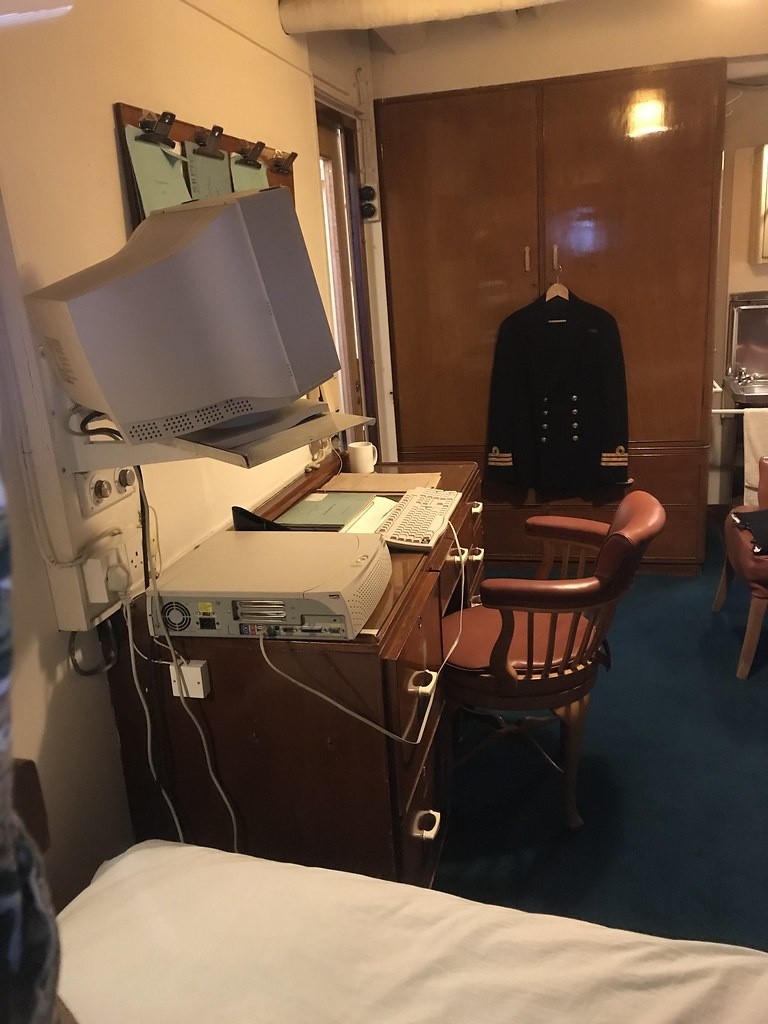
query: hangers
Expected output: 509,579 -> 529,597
546,266 -> 569,324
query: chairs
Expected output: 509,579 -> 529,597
711,454 -> 768,680
440,490 -> 667,829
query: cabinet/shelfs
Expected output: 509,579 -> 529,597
372,55 -> 729,580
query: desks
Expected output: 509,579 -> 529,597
97,463 -> 487,890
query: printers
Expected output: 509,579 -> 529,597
147,533 -> 395,641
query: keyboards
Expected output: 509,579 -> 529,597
374,487 -> 463,552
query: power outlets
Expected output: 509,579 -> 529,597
118,525 -> 157,584
81,542 -> 132,605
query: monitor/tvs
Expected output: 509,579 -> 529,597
19,184 -> 343,450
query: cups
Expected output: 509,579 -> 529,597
348,442 -> 378,473
